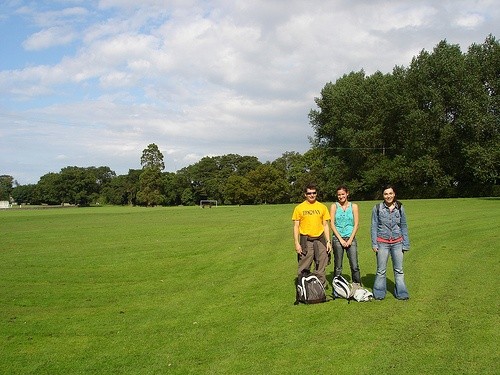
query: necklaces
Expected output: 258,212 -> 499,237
342,202 -> 348,212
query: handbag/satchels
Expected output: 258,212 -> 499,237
351,282 -> 374,302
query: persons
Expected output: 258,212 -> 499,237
329,186 -> 362,298
370,185 -> 410,300
292,186 -> 332,292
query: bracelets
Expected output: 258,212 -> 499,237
327,240 -> 331,244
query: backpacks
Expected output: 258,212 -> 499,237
293,268 -> 327,305
329,274 -> 353,304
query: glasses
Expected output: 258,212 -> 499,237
306,192 -> 316,195
